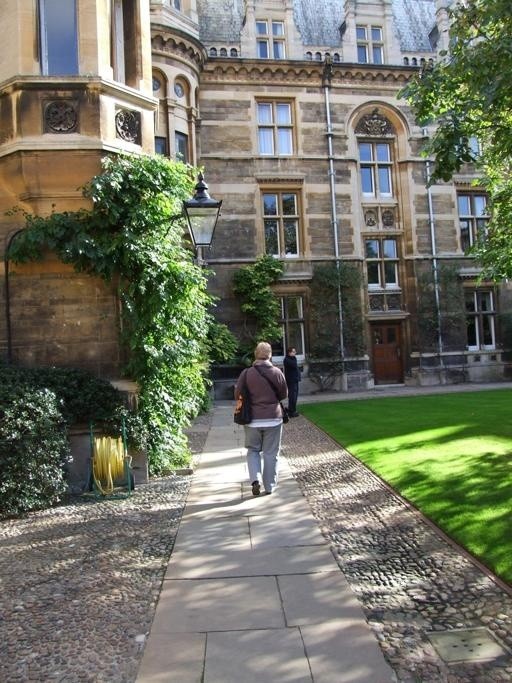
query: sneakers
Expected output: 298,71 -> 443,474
252,481 -> 260,495
266,492 -> 272,495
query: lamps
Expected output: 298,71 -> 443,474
126,170 -> 223,251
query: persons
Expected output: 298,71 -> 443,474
284,346 -> 302,418
234,341 -> 289,495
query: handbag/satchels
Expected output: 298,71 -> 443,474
281,407 -> 289,424
233,368 -> 251,425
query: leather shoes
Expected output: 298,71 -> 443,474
288,411 -> 299,418
